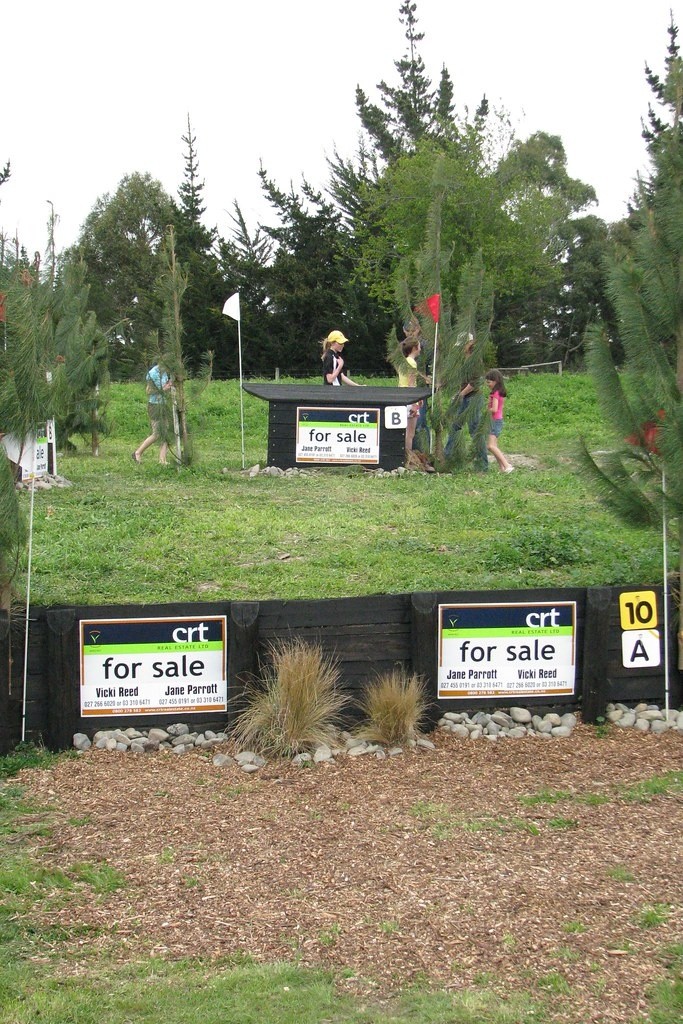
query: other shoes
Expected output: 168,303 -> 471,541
424,464 -> 435,472
132,452 -> 139,463
505,467 -> 514,475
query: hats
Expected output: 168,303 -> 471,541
454,332 -> 473,347
328,331 -> 348,344
404,317 -> 419,330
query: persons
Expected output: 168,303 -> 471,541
321,331 -> 367,387
397,318 -> 437,473
485,370 -> 514,475
442,332 -> 488,473
131,355 -> 175,467
